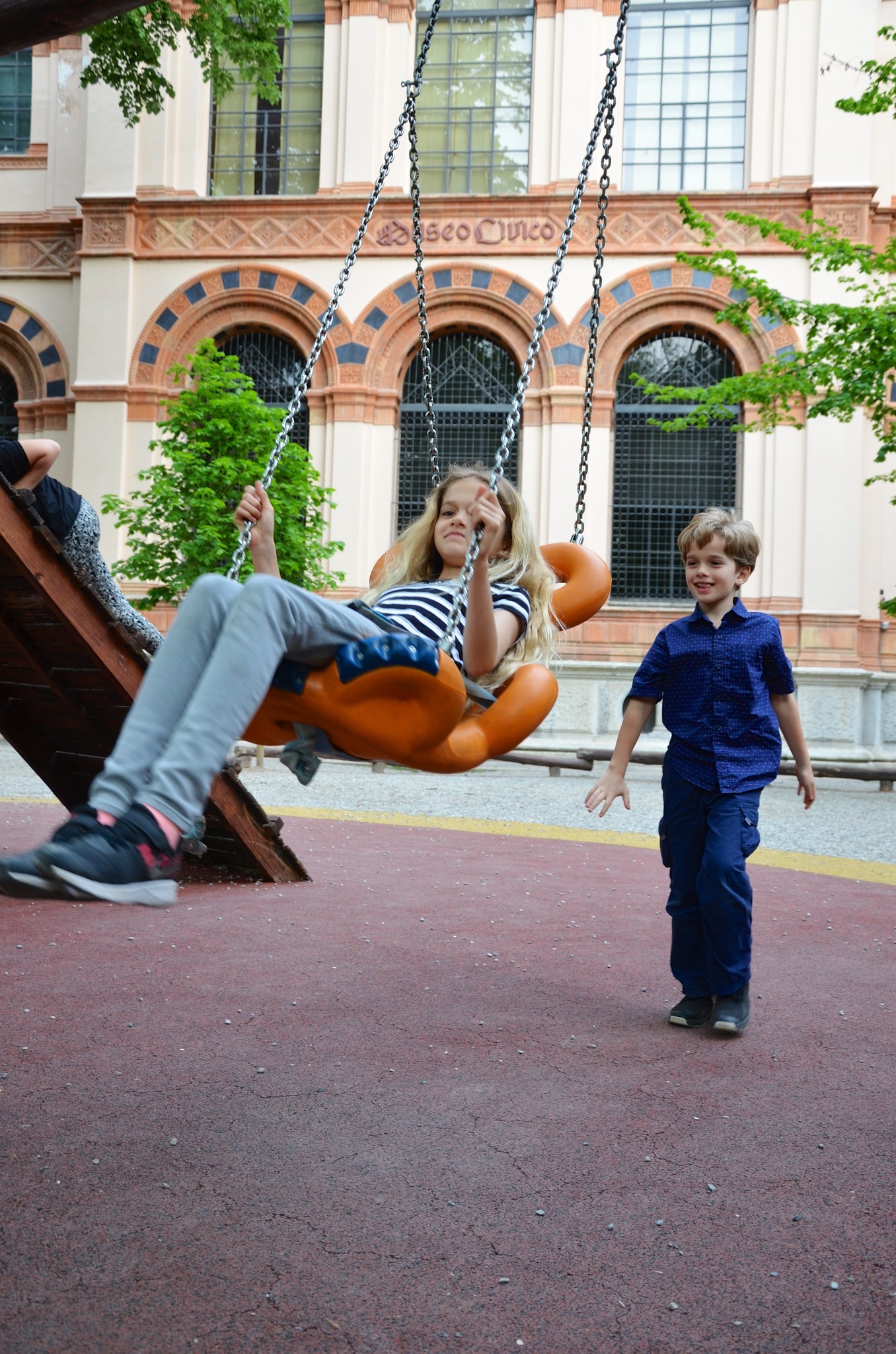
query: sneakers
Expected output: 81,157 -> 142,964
0,802 -> 104,899
49,804 -> 181,906
668,995 -> 717,1027
712,982 -> 750,1032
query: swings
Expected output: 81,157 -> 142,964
222,1 -> 631,776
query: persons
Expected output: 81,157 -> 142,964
584,506 -> 816,1031
0,439 -> 168,653
0,460 -> 556,908
